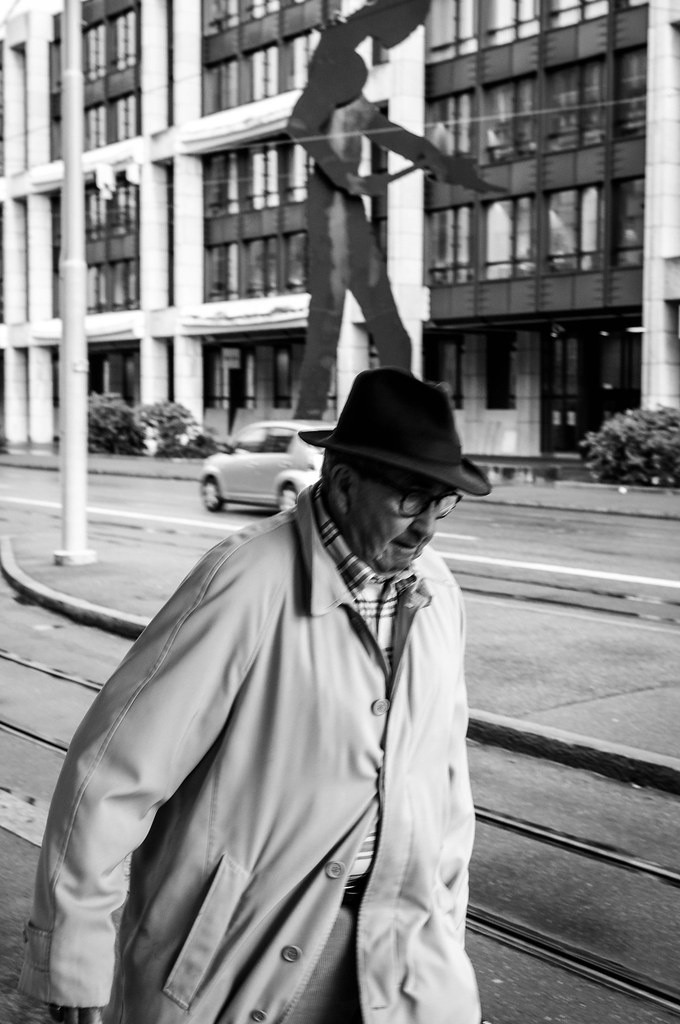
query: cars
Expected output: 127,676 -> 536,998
201,420 -> 338,517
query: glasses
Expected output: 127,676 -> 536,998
346,458 -> 463,519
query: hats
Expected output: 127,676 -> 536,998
299,365 -> 492,496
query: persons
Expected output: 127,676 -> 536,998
18,368 -> 490,1023
